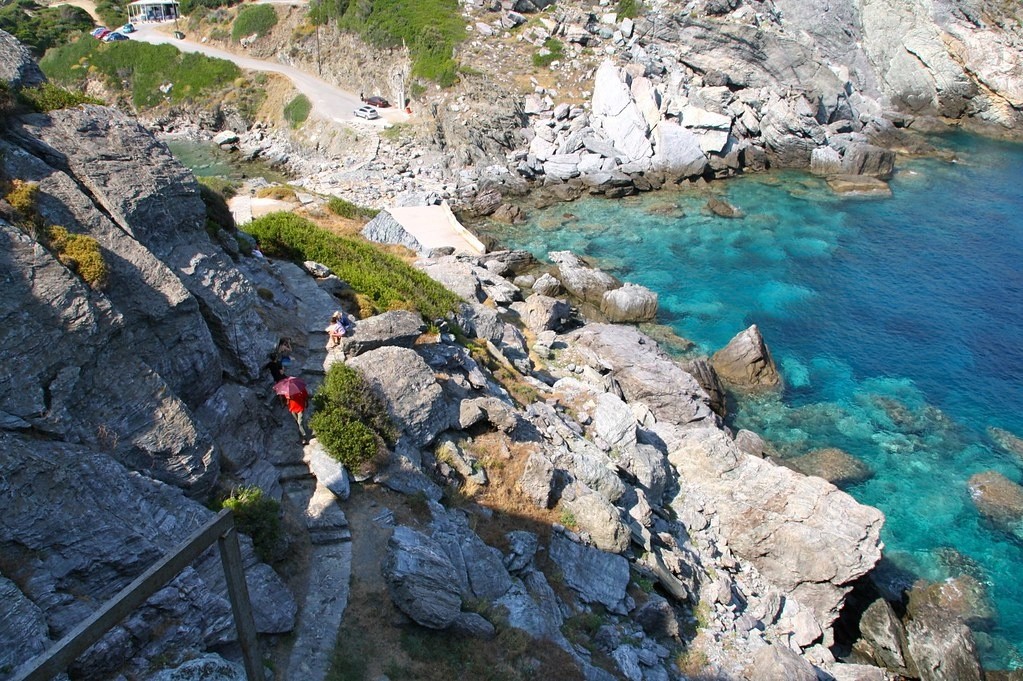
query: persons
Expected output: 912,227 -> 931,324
269,352 -> 286,384
360,91 -> 364,101
324,317 -> 346,348
275,334 -> 299,372
332,311 -> 356,336
284,386 -> 309,445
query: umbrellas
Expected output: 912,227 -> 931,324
272,375 -> 306,397
